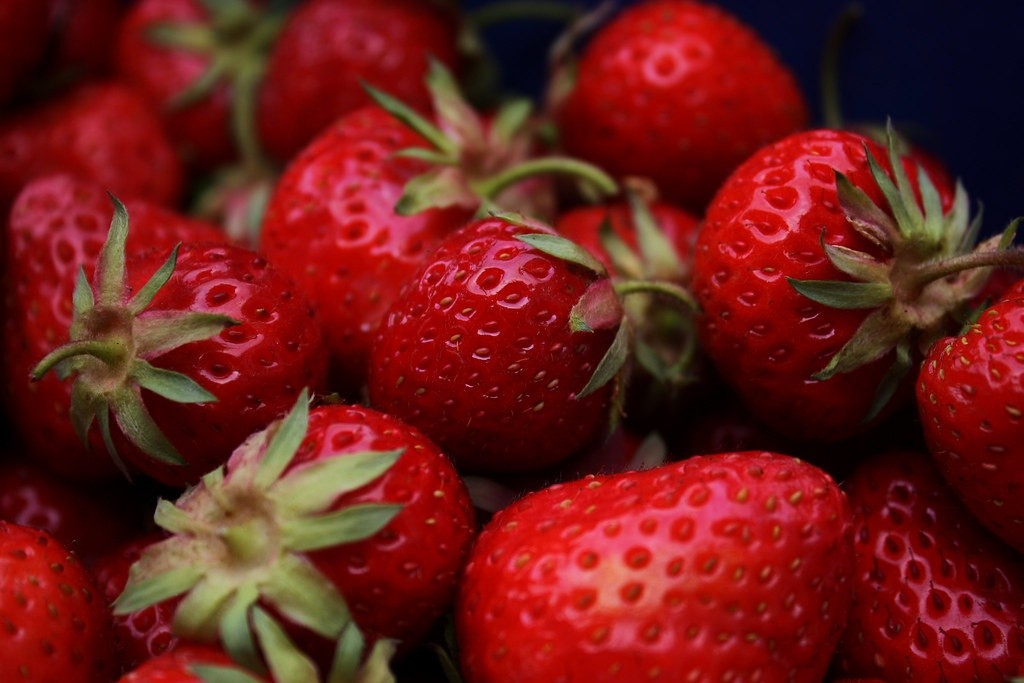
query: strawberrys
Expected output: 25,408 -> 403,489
3,2 -> 1024,683
